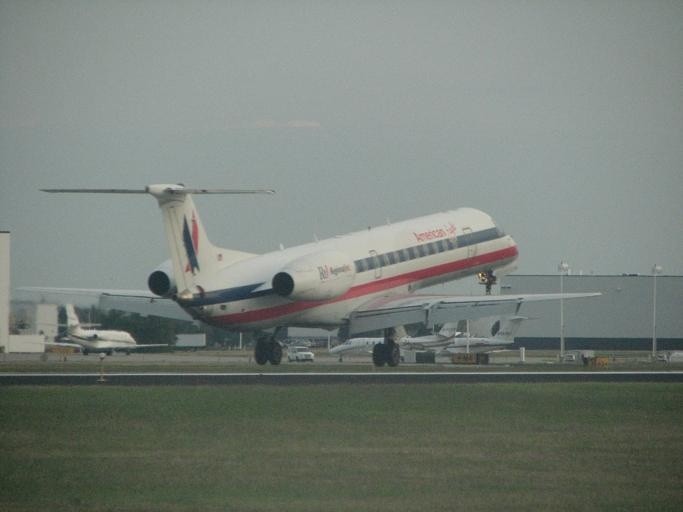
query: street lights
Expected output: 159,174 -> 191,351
557,259 -> 568,362
650,263 -> 662,358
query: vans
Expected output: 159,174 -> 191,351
286,345 -> 314,362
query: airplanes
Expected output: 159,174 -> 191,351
20,303 -> 168,360
329,311 -> 530,363
12,183 -> 603,365
